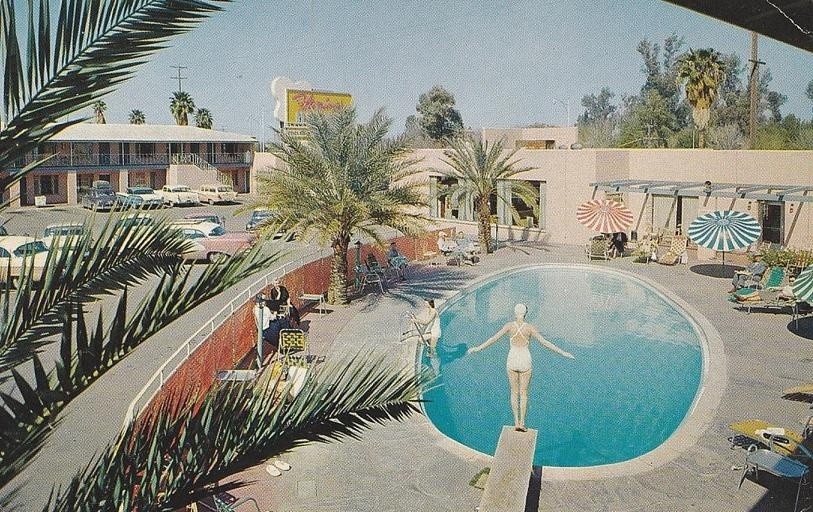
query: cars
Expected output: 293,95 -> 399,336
82,181 -> 123,212
43,223 -> 91,259
246,207 -> 292,240
0,236 -> 77,289
197,185 -> 237,205
164,219 -> 257,264
184,213 -> 226,226
114,213 -> 155,238
161,185 -> 199,206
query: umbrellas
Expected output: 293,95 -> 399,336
687,211 -> 762,265
792,263 -> 813,307
576,198 -> 635,233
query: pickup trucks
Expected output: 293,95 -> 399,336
117,187 -> 164,208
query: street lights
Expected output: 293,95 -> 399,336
354,241 -> 361,289
255,292 -> 264,367
494,216 -> 500,249
551,91 -> 571,126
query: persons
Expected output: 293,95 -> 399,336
408,298 -> 440,351
606,233 -> 628,258
467,302 -> 574,432
388,242 -> 408,281
728,252 -> 769,293
437,230 -> 480,266
253,277 -> 300,354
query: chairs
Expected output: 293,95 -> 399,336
733,265 -> 812,332
727,417 -> 812,511
586,232 -> 688,267
262,327 -> 309,400
